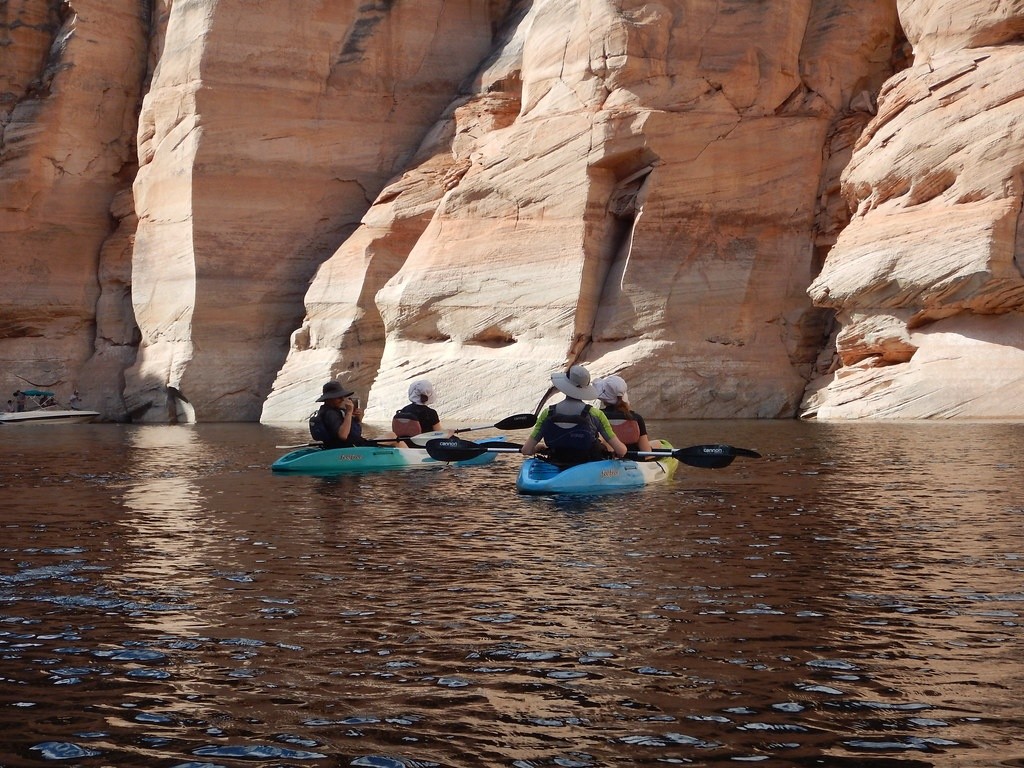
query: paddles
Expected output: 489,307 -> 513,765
275,428 -> 455,449
479,440 -> 763,459
425,437 -> 737,469
454,413 -> 538,434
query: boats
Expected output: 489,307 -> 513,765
272,435 -> 508,472
0,387 -> 102,426
513,440 -> 680,493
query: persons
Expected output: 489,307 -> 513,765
70,389 -> 83,410
16,390 -> 25,412
49,396 -> 57,405
592,376 -> 661,460
39,394 -> 49,408
309,381 -> 409,449
521,365 -> 627,470
7,399 -> 15,412
401,379 -> 459,440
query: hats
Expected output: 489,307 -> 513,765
593,375 -> 628,405
316,382 -> 354,402
408,380 -> 436,405
551,365 -> 599,400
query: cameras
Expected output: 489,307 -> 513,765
348,398 -> 360,409
